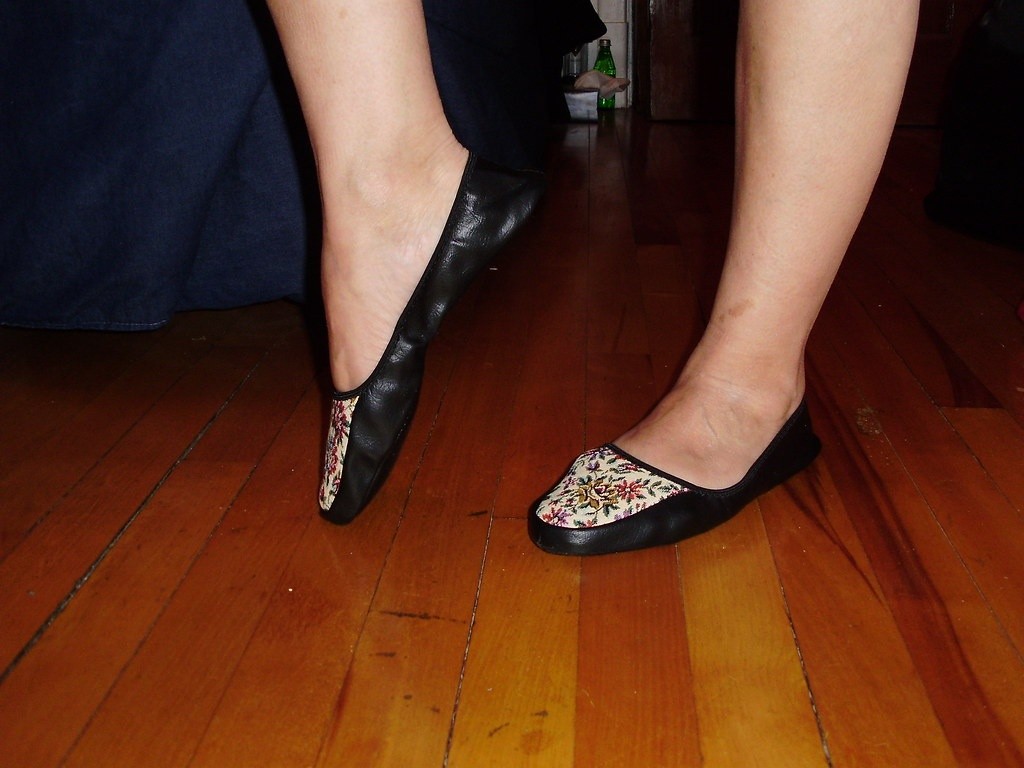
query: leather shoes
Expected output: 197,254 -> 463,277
529,396 -> 826,556
317,143 -> 550,524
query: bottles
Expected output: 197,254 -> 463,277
593,39 -> 617,110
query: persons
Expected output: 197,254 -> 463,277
263,0 -> 921,555
